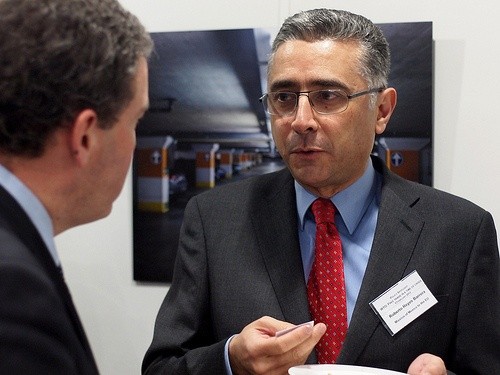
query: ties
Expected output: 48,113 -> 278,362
306,198 -> 347,364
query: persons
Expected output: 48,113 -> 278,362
140,6 -> 500,375
0,1 -> 153,375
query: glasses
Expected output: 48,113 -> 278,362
259,87 -> 384,116
